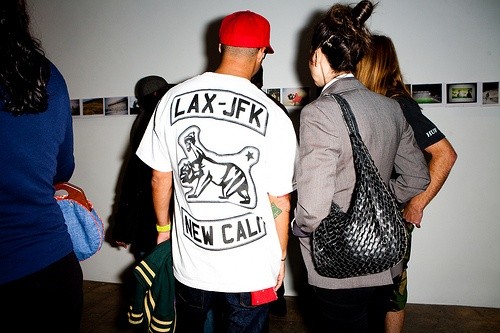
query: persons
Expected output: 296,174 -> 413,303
0,0 -> 84,333
104,0 -> 458,333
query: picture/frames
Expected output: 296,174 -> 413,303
262,87 -> 312,109
411,81 -> 500,107
70,96 -> 139,117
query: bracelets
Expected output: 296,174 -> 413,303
281,257 -> 286,261
156,223 -> 171,232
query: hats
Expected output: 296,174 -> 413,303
218,10 -> 274,54
134,76 -> 177,99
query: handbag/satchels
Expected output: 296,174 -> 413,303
53,182 -> 105,261
312,92 -> 411,279
